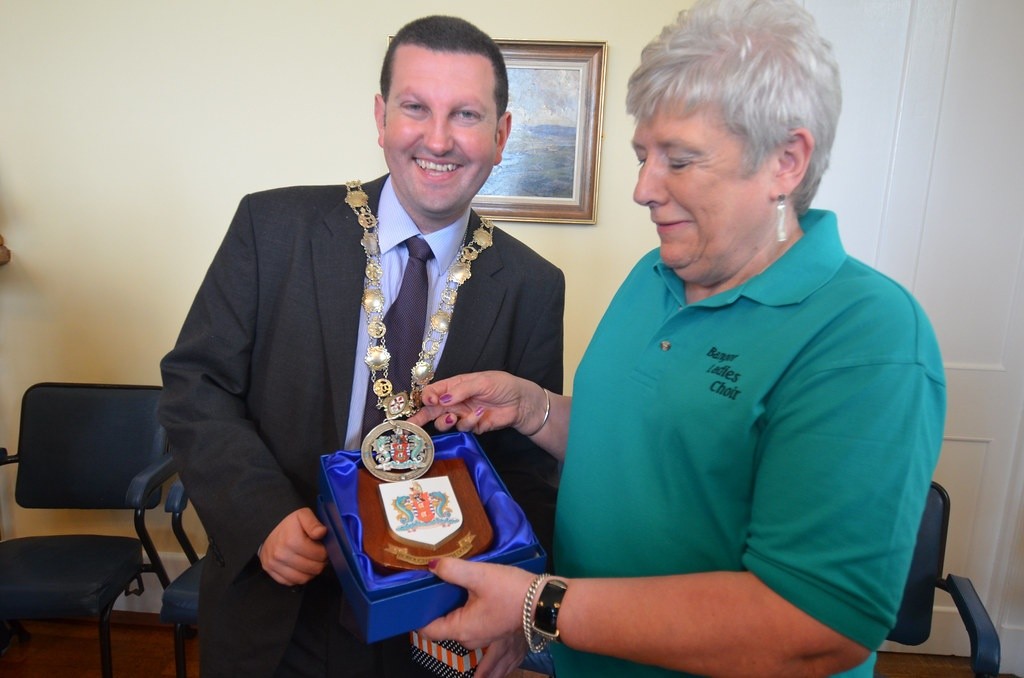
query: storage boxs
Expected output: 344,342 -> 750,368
408,630 -> 491,678
317,439 -> 550,645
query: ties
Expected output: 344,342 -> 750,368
361,236 -> 438,451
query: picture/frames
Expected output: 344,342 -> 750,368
461,40 -> 609,224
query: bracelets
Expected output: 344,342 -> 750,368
528,386 -> 550,438
523,573 -> 570,655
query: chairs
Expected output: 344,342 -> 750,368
1,381 -> 214,678
861,482 -> 1003,678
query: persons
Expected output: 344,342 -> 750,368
405,0 -> 948,678
159,14 -> 570,678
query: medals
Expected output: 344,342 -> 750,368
358,420 -> 436,481
